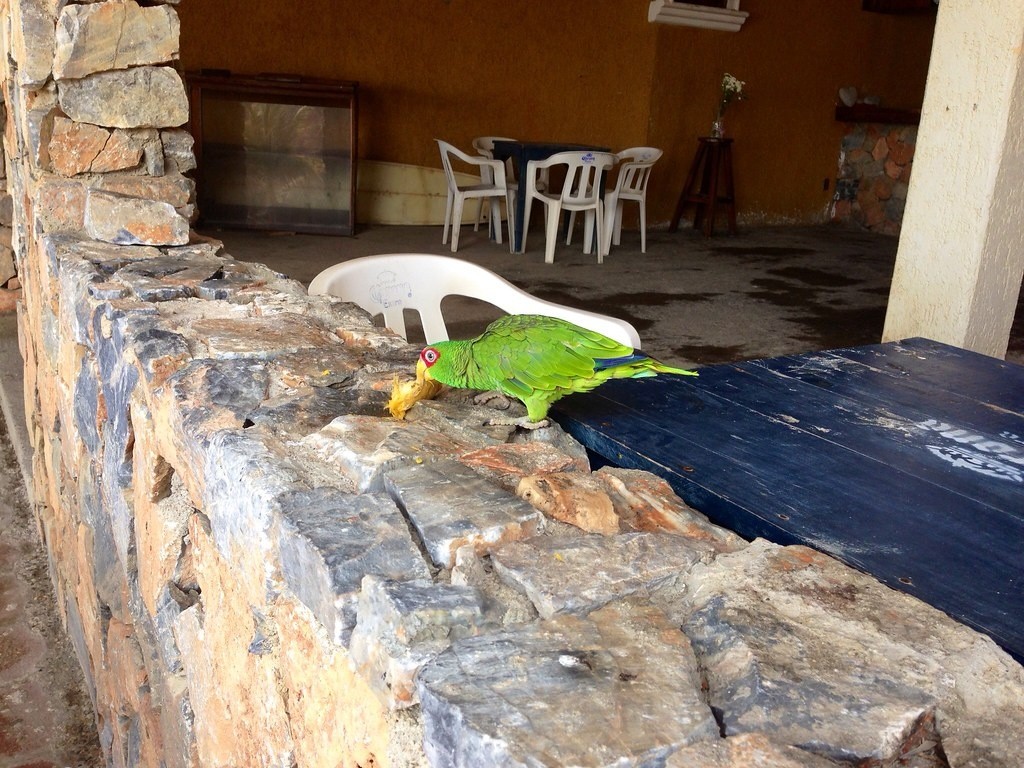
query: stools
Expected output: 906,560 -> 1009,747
668,137 -> 737,239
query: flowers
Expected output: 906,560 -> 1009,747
718,72 -> 749,118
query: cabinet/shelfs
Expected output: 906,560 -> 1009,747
178,69 -> 360,236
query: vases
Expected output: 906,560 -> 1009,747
711,110 -> 728,138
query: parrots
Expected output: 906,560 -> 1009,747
416,315 -> 700,429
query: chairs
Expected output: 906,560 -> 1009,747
306,253 -> 641,350
433,138 -> 515,254
520,151 -> 615,264
471,137 -> 549,239
566,146 -> 663,256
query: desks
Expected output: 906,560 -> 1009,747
547,338 -> 1024,667
490,140 -> 612,255
